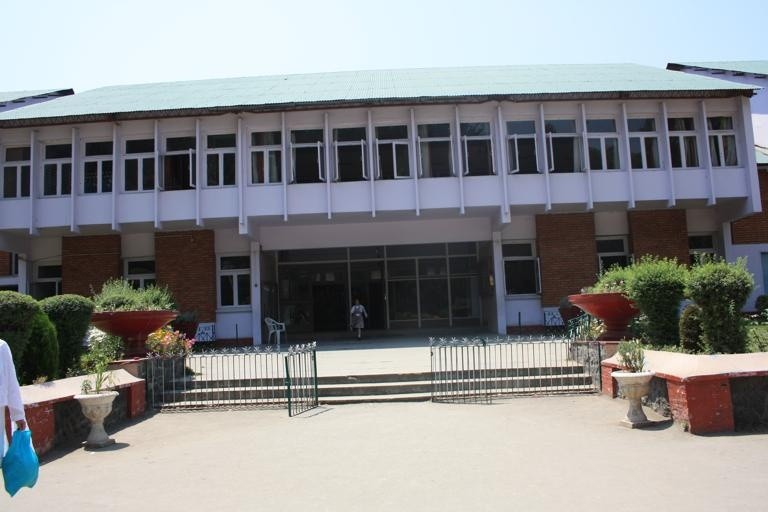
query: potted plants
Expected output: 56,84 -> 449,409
94,277 -> 181,358
567,280 -> 641,340
73,334 -> 126,448
610,338 -> 657,428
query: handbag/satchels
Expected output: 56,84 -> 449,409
1,428 -> 37,497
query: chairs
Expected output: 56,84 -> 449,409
264,317 -> 288,345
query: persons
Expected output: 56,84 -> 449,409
350,298 -> 368,342
0,337 -> 28,512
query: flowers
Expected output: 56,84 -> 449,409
145,326 -> 196,356
